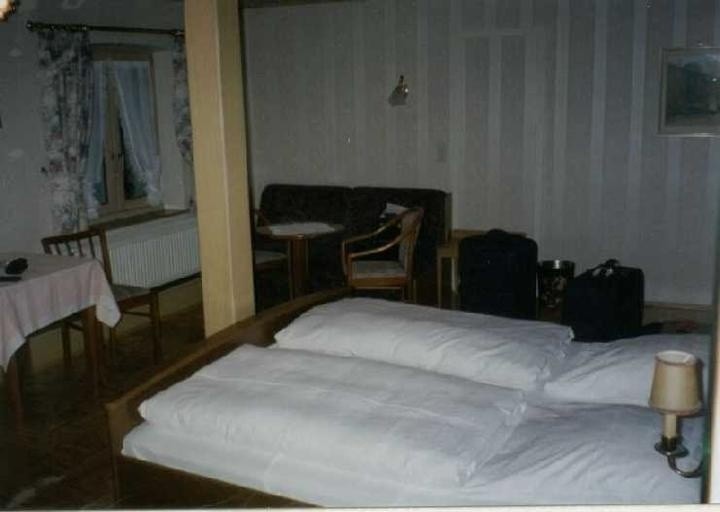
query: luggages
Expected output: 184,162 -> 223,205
458,228 -> 538,322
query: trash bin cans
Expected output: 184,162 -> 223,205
537,260 -> 574,308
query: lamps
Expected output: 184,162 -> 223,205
648,349 -> 704,478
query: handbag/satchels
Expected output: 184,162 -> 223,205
562,260 -> 644,343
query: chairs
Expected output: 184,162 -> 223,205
41,227 -> 162,373
341,207 -> 425,304
253,209 -> 288,272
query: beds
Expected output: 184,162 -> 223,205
100,286 -> 719,509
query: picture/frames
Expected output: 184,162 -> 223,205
657,46 -> 720,138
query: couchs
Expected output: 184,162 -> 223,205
256,184 -> 446,289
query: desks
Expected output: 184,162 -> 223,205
0,251 -> 100,448
255,221 -> 347,301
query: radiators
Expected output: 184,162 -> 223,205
52,213 -> 201,291
